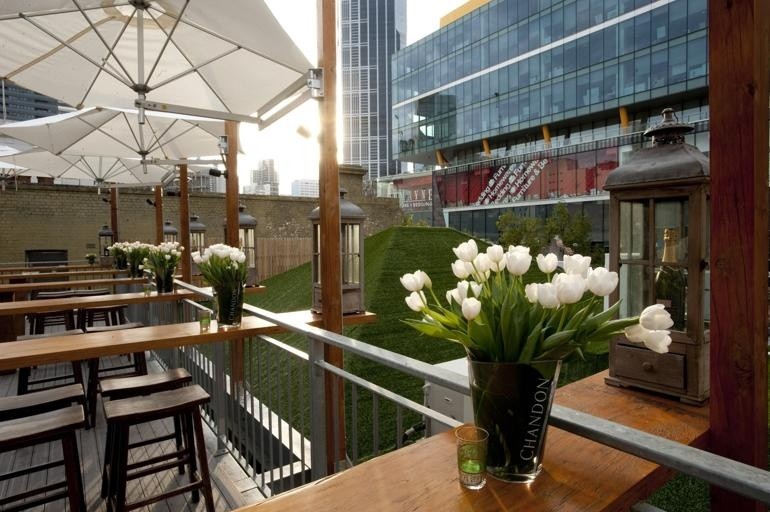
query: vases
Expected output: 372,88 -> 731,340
114,257 -> 128,270
155,269 -> 174,294
130,259 -> 144,279
218,286 -> 242,325
467,360 -> 561,485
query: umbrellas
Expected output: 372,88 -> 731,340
0,107 -> 245,174
0,148 -> 194,195
0,0 -> 314,124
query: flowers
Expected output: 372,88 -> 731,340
191,244 -> 250,324
138,242 -> 184,293
120,241 -> 154,277
400,239 -> 673,473
107,242 -> 130,269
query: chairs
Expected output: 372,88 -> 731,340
0,404 -> 87,512
99,367 -> 192,503
82,303 -> 131,362
35,293 -> 73,335
100,385 -> 216,512
0,384 -> 85,506
17,328 -> 84,393
17,361 -> 90,432
85,321 -> 147,427
26,310 -> 75,376
74,291 -> 109,330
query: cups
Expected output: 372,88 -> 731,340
199,309 -> 210,331
455,426 -> 489,490
142,283 -> 152,296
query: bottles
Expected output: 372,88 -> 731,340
654,228 -> 685,332
